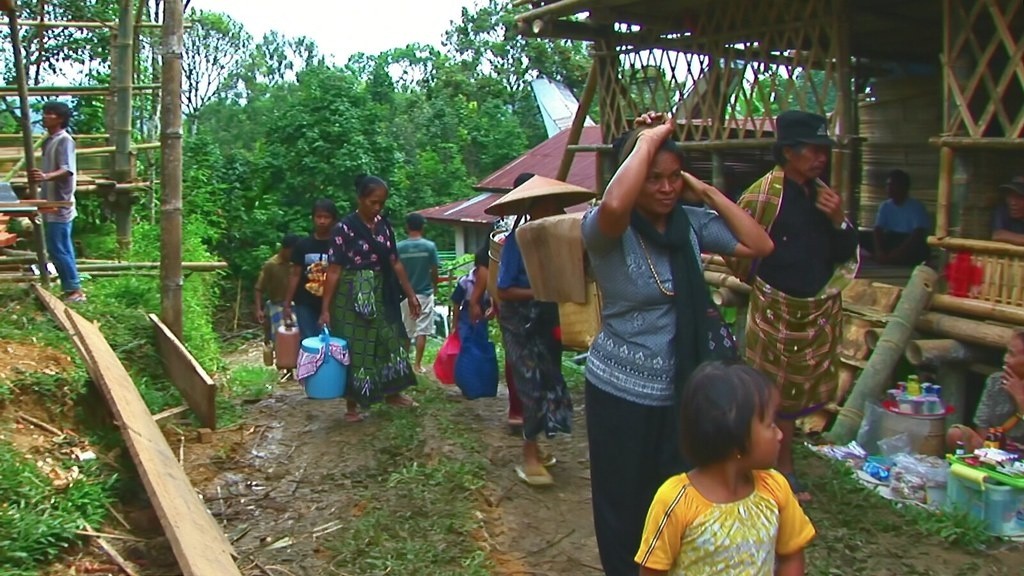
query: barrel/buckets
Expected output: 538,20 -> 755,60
301,323 -> 349,400
879,401 -> 947,459
274,317 -> 300,370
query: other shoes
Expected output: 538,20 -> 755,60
343,410 -> 366,422
386,397 -> 420,409
507,415 -> 524,426
67,294 -> 86,302
515,464 -> 554,485
538,449 -> 557,467
415,367 -> 427,373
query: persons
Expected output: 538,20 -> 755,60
634,360 -> 816,575
990,173 -> 1024,245
450,171 -> 599,487
395,214 -> 439,374
946,325 -> 1024,452
857,169 -> 932,267
253,235 -> 299,380
715,109 -> 860,505
284,199 -> 338,351
582,110 -> 774,576
317,172 -> 421,423
27,102 -> 86,303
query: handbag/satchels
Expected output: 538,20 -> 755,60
433,325 -> 460,384
305,262 -> 328,296
263,345 -> 273,365
352,278 -> 378,320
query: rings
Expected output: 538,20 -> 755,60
1003,380 -> 1006,384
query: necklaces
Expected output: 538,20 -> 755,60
634,225 -> 675,297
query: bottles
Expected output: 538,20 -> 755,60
262,340 -> 273,366
886,375 -> 943,413
953,441 -> 966,455
982,425 -> 1008,451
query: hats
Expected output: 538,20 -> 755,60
776,110 -> 837,146
1000,176 -> 1024,196
484,174 -> 596,216
